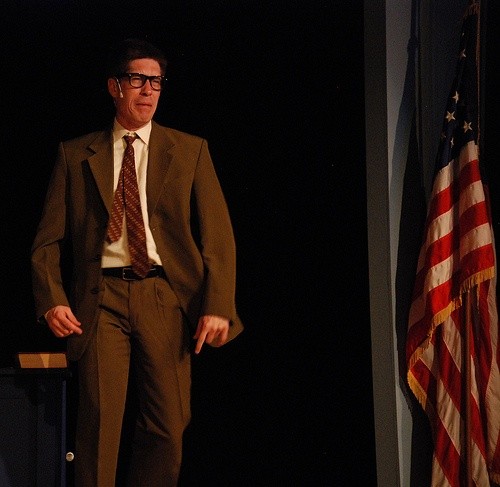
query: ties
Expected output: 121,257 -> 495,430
106,136 -> 149,278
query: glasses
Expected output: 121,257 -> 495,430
119,73 -> 167,90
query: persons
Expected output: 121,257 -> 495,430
30,38 -> 244,487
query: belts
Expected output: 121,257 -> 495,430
103,268 -> 162,281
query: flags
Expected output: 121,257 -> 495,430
404,0 -> 500,487
